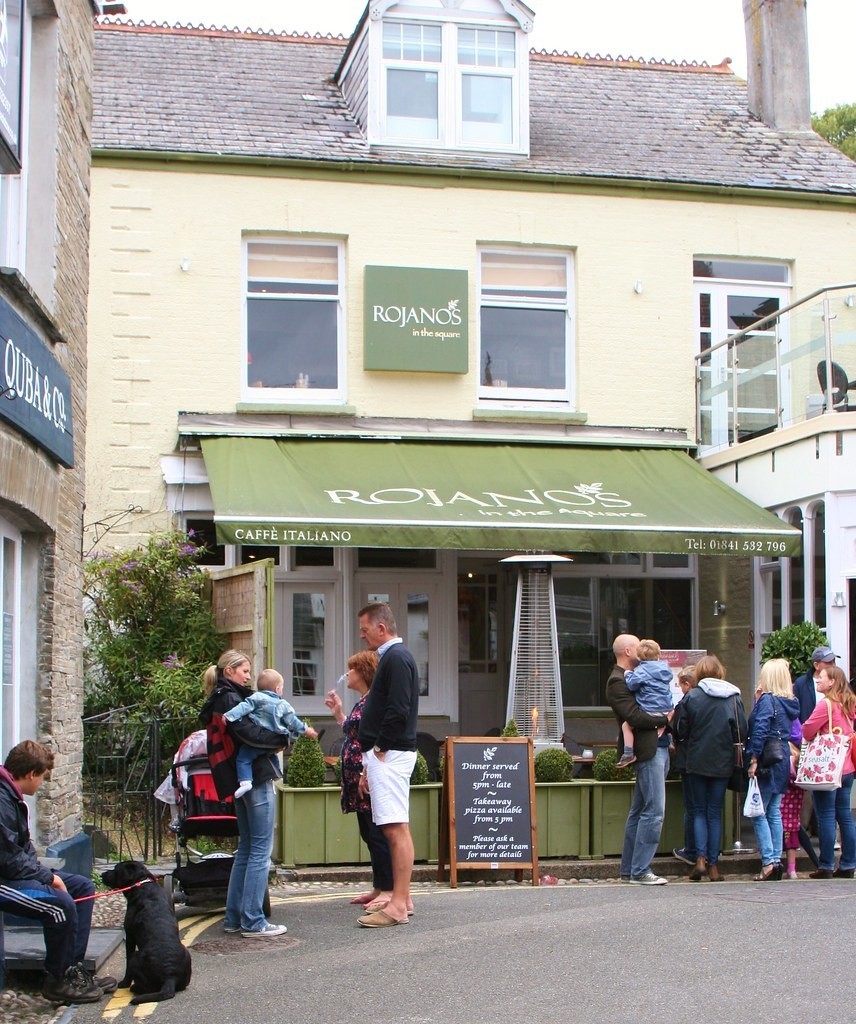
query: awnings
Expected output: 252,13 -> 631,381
199,436 -> 802,558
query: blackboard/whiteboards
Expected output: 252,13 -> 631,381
439,736 -> 539,869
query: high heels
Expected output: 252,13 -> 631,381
752,863 -> 784,880
833,864 -> 854,879
809,868 -> 834,879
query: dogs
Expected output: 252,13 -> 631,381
100,860 -> 193,1006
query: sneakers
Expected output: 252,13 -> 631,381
630,870 -> 668,885
71,963 -> 117,993
621,874 -> 629,883
615,754 -> 637,769
673,848 -> 696,866
41,966 -> 104,1004
223,923 -> 243,933
667,744 -> 676,757
242,922 -> 287,937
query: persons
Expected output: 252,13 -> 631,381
357,601 -> 418,928
325,650 -> 394,910
199,650 -> 319,937
606,632 -> 856,884
1,740 -> 118,1003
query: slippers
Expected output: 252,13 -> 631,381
362,898 -> 385,910
366,901 -> 414,916
350,892 -> 372,905
357,908 -> 411,927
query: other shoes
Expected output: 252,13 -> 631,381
708,865 -> 725,880
783,871 -> 798,881
689,858 -> 707,881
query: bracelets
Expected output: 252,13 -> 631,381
374,745 -> 387,753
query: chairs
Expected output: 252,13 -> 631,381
417,732 -> 440,782
485,728 -> 501,736
817,360 -> 856,412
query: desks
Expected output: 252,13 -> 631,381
323,757 -> 339,767
570,754 -> 596,778
578,742 -> 619,748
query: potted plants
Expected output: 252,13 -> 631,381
534,748 -> 723,860
760,619 -> 831,828
275,717 -> 442,868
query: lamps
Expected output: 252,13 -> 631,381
634,280 -> 645,296
832,592 -> 846,607
713,601 -> 725,616
845,293 -> 855,308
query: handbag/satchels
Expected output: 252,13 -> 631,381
742,777 -> 764,817
732,743 -> 744,769
761,738 -> 783,766
794,726 -> 851,791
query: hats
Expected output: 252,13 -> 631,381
811,646 -> 841,666
